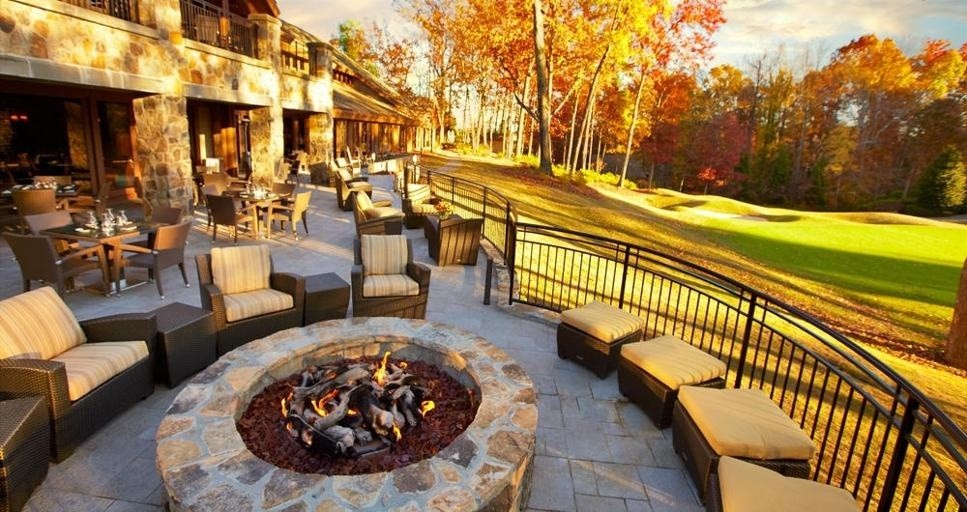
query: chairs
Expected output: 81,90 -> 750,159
3,174 -> 192,300
0,312 -> 158,462
196,149 -> 484,268
194,252 -> 306,358
350,240 -> 432,320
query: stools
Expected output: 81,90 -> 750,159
705,455 -> 860,512
556,299 -> 644,380
671,385 -> 817,509
618,334 -> 728,429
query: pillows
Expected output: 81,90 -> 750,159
212,244 -> 270,295
0,285 -> 87,360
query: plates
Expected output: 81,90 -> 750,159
79,229 -> 91,234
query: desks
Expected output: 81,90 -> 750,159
0,396 -> 50,512
303,271 -> 350,328
148,302 -> 218,389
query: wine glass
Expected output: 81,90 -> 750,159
27,178 -> 76,192
238,184 -> 272,194
87,207 -> 129,229
